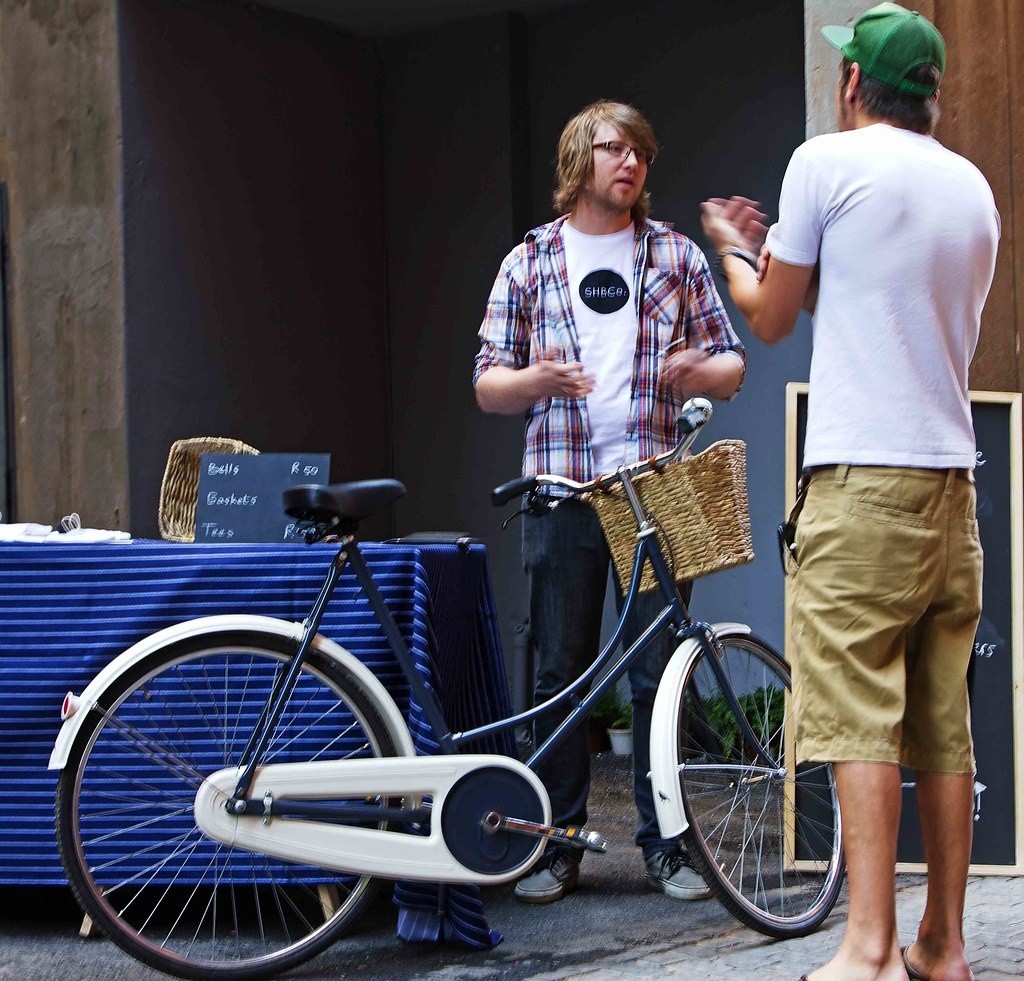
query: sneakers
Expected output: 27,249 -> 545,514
514,845 -> 581,903
645,846 -> 713,901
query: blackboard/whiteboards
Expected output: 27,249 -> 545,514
191,449 -> 332,544
786,374 -> 1024,879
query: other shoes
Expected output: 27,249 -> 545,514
799,945 -> 963,980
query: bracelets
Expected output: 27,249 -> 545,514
713,247 -> 758,281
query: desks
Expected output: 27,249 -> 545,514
0,541 -> 511,936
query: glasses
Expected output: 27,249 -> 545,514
591,140 -> 655,166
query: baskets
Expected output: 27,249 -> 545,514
592,439 -> 754,596
160,438 -> 260,544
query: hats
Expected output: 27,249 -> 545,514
820,2 -> 947,97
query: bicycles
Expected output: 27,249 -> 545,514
48,399 -> 845,981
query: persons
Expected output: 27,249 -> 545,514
699,4 -> 1004,980
476,102 -> 750,904
